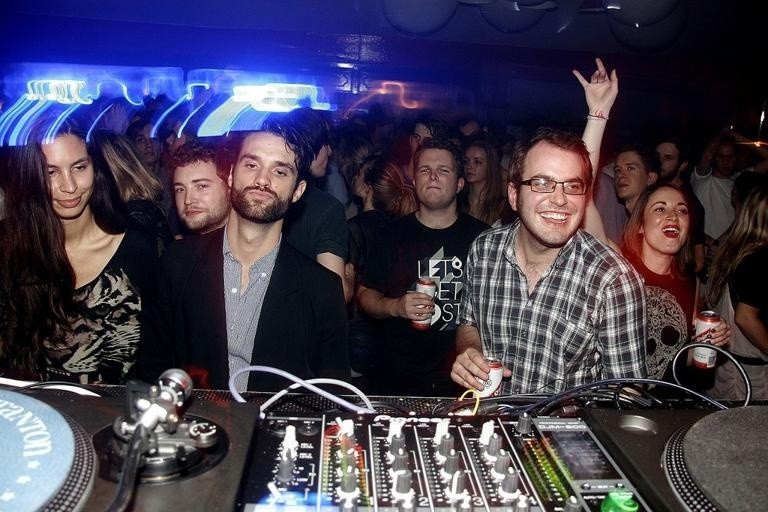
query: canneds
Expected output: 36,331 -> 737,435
472,357 -> 503,402
412,280 -> 436,330
694,310 -> 721,369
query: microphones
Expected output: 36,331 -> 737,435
124,368 -> 193,439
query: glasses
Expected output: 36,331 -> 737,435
518,178 -> 590,196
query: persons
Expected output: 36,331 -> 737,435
1,57 -> 767,400
